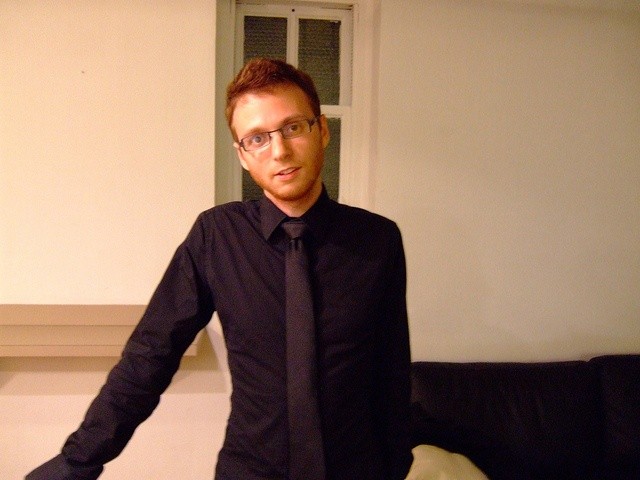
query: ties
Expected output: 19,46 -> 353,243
280,220 -> 327,478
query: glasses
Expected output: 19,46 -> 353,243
236,118 -> 322,152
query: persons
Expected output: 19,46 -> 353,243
23,57 -> 414,480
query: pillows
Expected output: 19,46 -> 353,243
405,443 -> 488,478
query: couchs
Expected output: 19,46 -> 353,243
412,356 -> 640,479
